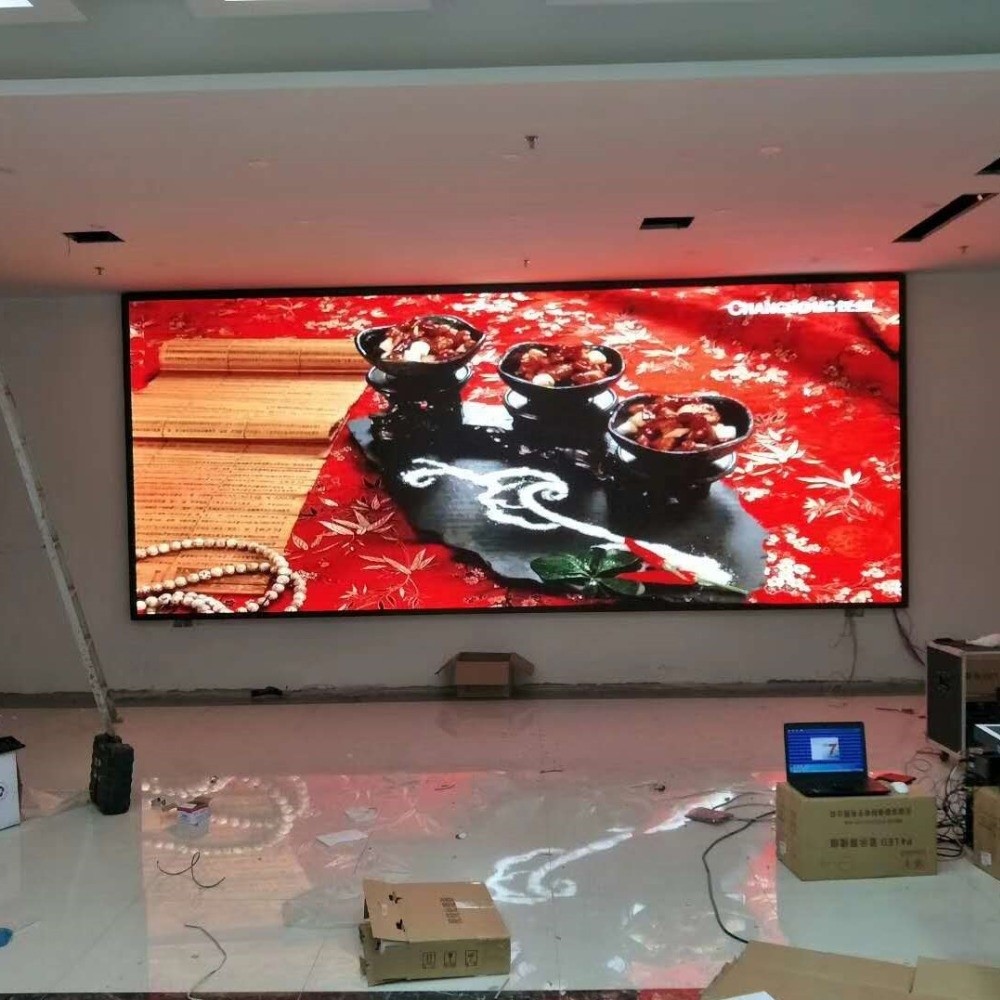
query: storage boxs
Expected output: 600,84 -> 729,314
89,732 -> 134,816
973,787 -> 1000,880
358,878 -> 511,987
433,651 -> 534,698
774,780 -> 937,881
926,641 -> 1000,762
178,801 -> 209,820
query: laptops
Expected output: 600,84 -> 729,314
783,721 -> 890,797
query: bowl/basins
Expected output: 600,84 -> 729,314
355,314 -> 486,382
496,341 -> 626,406
607,393 -> 754,470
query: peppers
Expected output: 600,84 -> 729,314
611,536 -> 748,595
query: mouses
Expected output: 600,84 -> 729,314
891,782 -> 909,793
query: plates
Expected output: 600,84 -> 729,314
365,363 -> 472,397
616,444 -> 737,485
504,387 -> 618,427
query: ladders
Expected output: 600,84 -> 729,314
0,383 -> 119,736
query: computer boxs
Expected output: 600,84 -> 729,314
965,750 -> 1000,849
